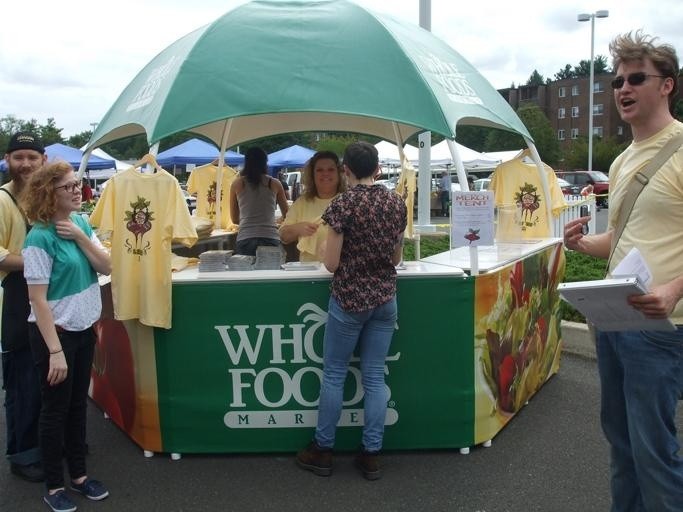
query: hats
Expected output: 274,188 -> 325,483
6,132 -> 45,154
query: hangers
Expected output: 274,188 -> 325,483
135,153 -> 160,172
519,148 -> 535,164
212,158 -> 228,168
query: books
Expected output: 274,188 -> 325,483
556,246 -> 678,333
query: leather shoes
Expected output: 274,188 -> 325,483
364,450 -> 381,480
297,444 -> 333,475
10,463 -> 44,482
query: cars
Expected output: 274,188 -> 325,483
376,167 -> 494,213
183,189 -> 197,214
92,179 -> 106,207
556,176 -> 580,195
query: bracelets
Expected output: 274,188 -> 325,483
49,349 -> 63,355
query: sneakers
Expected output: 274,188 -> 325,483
44,489 -> 77,512
70,478 -> 109,500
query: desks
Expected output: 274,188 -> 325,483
170,257 -> 473,461
421,236 -> 565,447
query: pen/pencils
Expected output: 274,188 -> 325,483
317,219 -> 324,225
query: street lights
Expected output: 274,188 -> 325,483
577,9 -> 608,171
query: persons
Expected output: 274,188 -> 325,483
277,171 -> 290,200
228,146 -> 290,256
295,139 -> 409,482
0,130 -> 49,483
81,176 -> 92,202
560,27 -> 682,512
19,159 -> 113,512
278,150 -> 347,264
436,171 -> 449,217
586,180 -> 600,211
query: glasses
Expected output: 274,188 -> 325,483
56,180 -> 82,192
611,75 -> 666,88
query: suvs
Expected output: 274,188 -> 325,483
554,170 -> 610,209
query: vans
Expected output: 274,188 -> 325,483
281,171 -> 303,200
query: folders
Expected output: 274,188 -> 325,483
557,277 -> 678,333
